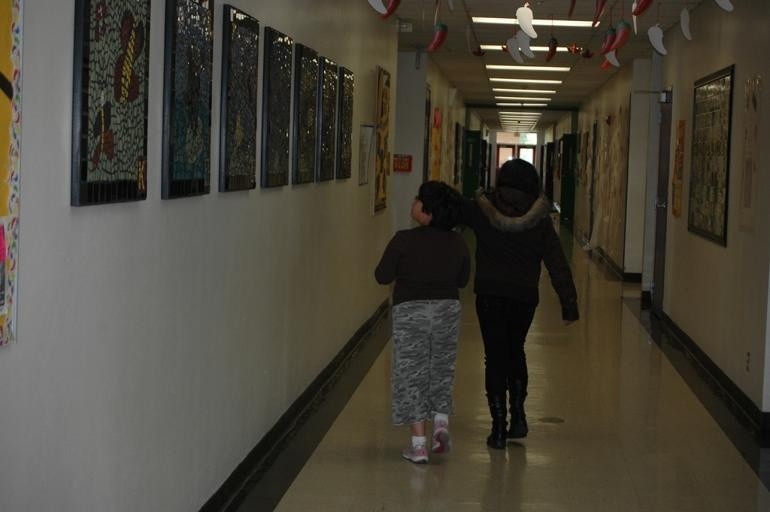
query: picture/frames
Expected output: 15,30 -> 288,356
161,1 -> 214,200
423,82 -> 462,185
260,26 -> 294,189
218,3 -> 260,193
687,63 -> 735,249
360,125 -> 372,183
292,42 -> 355,185
374,66 -> 391,213
0,0 -> 22,348
70,1 -> 152,207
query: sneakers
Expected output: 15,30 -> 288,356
432,427 -> 450,453
402,447 -> 429,464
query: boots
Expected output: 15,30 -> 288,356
486,378 -> 528,448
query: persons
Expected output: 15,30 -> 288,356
460,159 -> 581,449
374,180 -> 471,463
